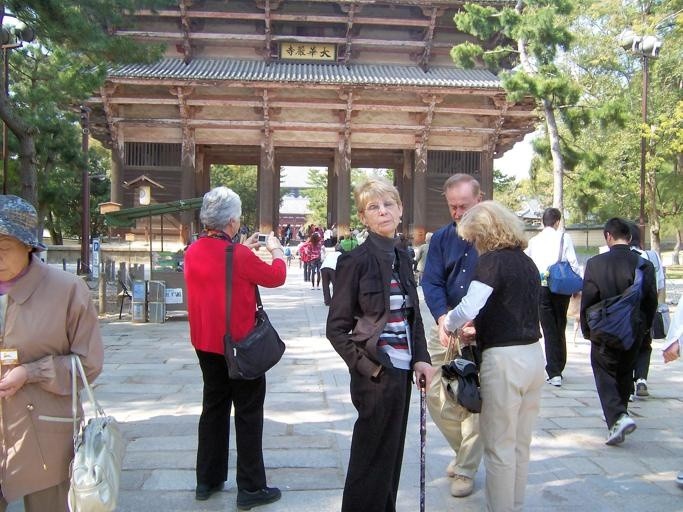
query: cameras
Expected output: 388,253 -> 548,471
257,235 -> 269,246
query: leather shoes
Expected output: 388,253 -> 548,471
194,478 -> 225,501
236,487 -> 282,510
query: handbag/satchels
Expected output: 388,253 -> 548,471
546,231 -> 584,296
651,303 -> 672,339
66,354 -> 122,512
218,243 -> 286,380
585,255 -> 647,354
438,328 -> 471,423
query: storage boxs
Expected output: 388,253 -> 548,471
131,302 -> 147,322
133,280 -> 147,302
147,302 -> 166,323
146,280 -> 165,302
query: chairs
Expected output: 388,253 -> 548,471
117,280 -> 132,318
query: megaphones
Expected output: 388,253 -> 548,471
0,25 -> 12,44
641,35 -> 662,53
620,29 -> 640,51
15,22 -> 36,42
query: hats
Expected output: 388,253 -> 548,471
0,194 -> 48,254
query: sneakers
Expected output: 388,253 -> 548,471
603,414 -> 639,446
447,457 -> 475,498
311,287 -> 321,290
545,376 -> 563,388
636,377 -> 650,397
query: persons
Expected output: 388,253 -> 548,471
628,220 -> 666,403
521,207 -> 579,386
231,223 -> 434,305
0,190 -> 103,512
662,293 -> 683,482
440,199 -> 546,511
415,173 -> 487,497
581,216 -> 658,444
324,172 -> 435,512
183,186 -> 286,510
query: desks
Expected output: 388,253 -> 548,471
151,271 -> 188,319
